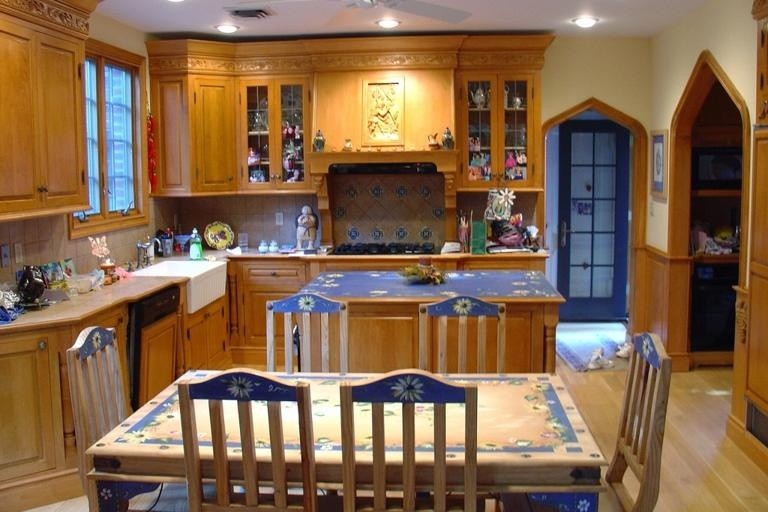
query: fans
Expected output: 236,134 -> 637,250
347,0 -> 471,24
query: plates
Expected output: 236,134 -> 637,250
203,221 -> 234,251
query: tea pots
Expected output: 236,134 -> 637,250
470,88 -> 488,110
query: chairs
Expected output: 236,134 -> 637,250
67,326 -> 233,511
342,369 -> 477,510
266,293 -> 347,373
177,368 -> 317,511
418,295 -> 506,374
597,332 -> 669,511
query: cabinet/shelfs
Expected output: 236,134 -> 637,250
239,58 -> 309,192
0,0 -> 97,223
457,51 -> 543,188
150,56 -> 234,192
186,292 -> 233,370
689,128 -> 742,366
226,259 -> 305,362
0,304 -> 130,512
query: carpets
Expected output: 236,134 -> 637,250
557,322 -> 634,371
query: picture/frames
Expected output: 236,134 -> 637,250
647,129 -> 668,201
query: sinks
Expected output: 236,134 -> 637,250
130,259 -> 227,315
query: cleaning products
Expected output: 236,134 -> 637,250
189,227 -> 203,259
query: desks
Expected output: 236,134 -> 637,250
85,370 -> 608,510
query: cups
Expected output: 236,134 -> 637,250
75,279 -> 92,294
512,97 -> 523,110
237,232 -> 248,253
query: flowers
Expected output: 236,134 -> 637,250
484,187 -> 515,221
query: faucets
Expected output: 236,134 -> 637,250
135,237 -> 163,268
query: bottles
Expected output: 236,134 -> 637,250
269,240 -> 279,252
188,228 -> 203,260
161,233 -> 173,257
257,240 -> 269,252
313,129 -> 326,151
344,138 -> 352,150
